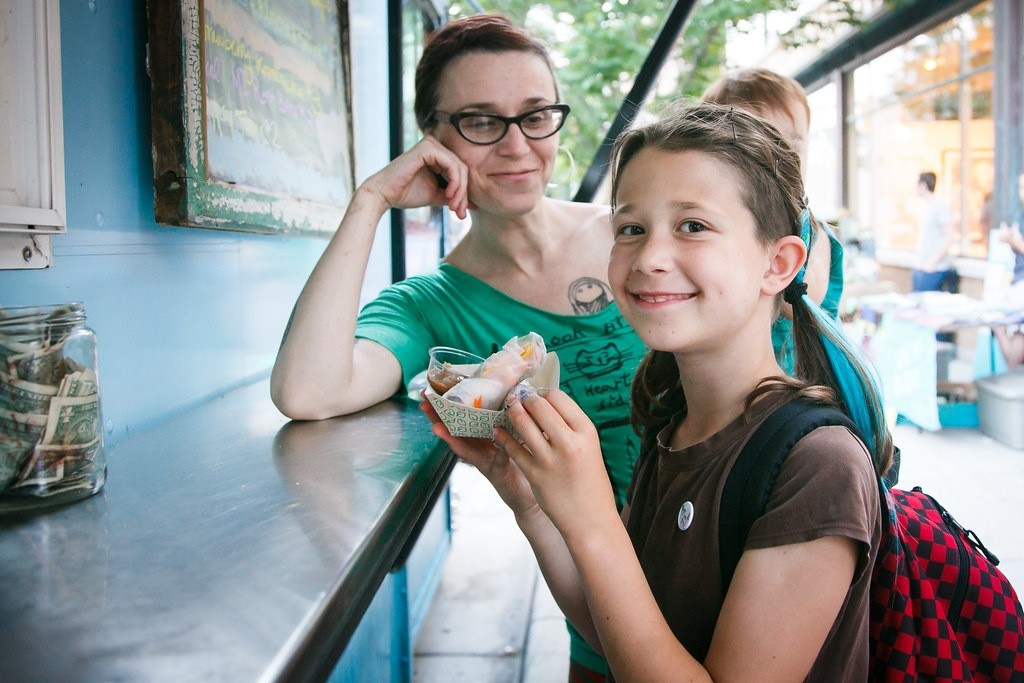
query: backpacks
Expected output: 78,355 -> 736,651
718,396 -> 1024,683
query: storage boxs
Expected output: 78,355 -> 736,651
971,366 -> 1024,450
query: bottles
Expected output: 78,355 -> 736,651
0,301 -> 107,512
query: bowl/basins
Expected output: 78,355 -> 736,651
427,346 -> 487,392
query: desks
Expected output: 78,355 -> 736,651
847,290 -> 1024,434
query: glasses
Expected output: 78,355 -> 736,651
430,104 -> 571,144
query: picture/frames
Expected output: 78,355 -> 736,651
142,0 -> 360,237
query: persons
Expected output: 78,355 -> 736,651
912,172 -> 959,341
421,105 -> 881,683
270,15 -> 654,683
703,70 -> 845,377
991,172 -> 1024,371
